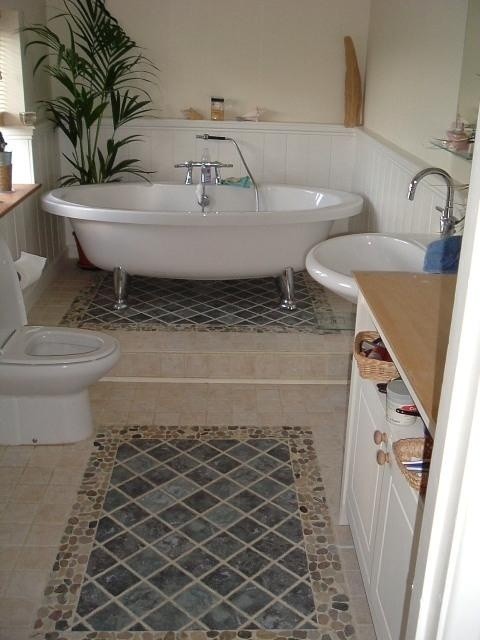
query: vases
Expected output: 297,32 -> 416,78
16,1 -> 160,271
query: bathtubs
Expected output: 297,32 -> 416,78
38,181 -> 363,281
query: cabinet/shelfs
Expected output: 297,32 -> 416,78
339,270 -> 457,640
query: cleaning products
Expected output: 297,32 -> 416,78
200,144 -> 212,184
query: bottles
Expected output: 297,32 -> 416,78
0,152 -> 13,192
385,379 -> 418,427
210,95 -> 225,121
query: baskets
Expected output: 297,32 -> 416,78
393,437 -> 433,493
354,331 -> 400,381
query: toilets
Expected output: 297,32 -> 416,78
0,233 -> 122,447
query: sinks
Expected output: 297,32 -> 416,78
305,233 -> 446,304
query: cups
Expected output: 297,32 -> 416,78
19,111 -> 37,127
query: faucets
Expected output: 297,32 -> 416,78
407,167 -> 459,240
